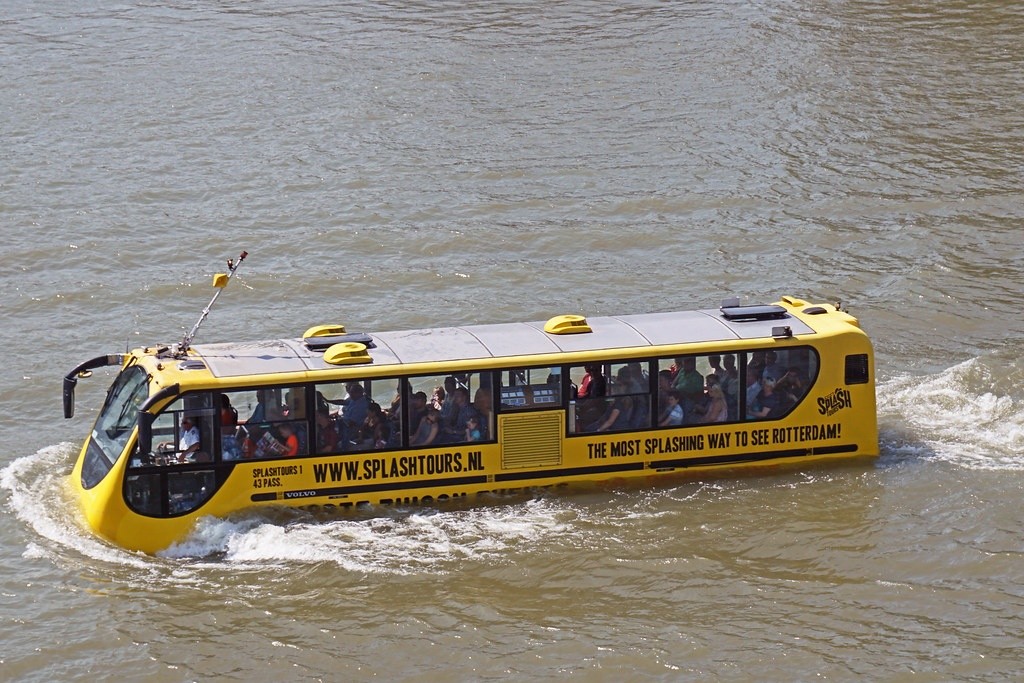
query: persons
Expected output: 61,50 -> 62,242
157,417 -> 200,464
220,388 -> 298,459
667,350 -> 807,425
547,360 -> 684,432
317,377 -> 481,452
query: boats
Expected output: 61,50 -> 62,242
64,251 -> 880,553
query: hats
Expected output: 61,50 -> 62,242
411,391 -> 427,401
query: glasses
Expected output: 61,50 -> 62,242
426,417 -> 434,424
590,368 -> 599,372
767,376 -> 774,381
182,421 -> 187,424
